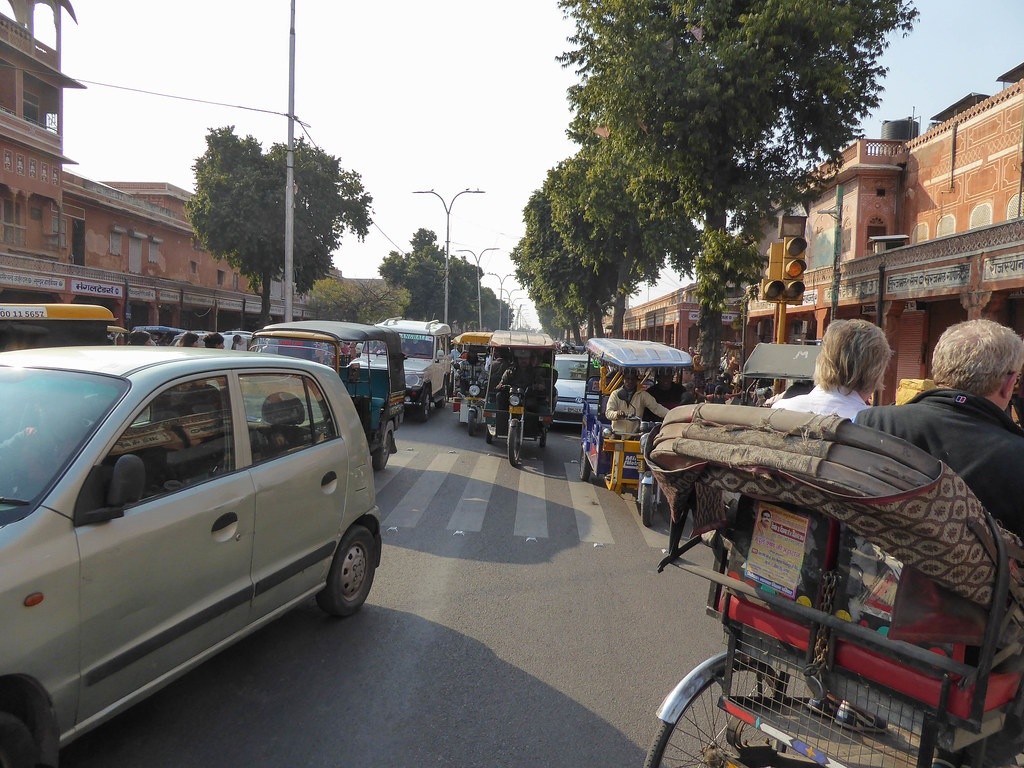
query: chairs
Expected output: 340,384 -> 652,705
138,384 -> 225,486
251,391 -> 322,462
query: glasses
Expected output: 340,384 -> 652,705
1007,369 -> 1023,386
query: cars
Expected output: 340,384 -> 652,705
101,327 -> 264,350
554,354 -> 599,432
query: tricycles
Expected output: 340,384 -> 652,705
451,331 -> 560,466
642,401 -> 1024,768
246,320 -> 407,471
578,337 -> 697,527
0,304 -> 117,352
740,343 -> 823,406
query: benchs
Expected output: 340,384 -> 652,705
582,375 -> 611,451
642,402 -> 1024,753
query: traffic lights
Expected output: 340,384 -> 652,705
759,237 -> 807,306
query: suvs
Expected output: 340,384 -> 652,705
0,347 -> 382,768
345,316 -> 452,423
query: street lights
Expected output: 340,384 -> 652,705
413,189 -> 486,325
487,273 -> 516,330
497,287 -> 529,331
456,248 -> 501,331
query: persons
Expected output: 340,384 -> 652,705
684,367 -> 785,407
496,348 -> 547,411
771,320 -> 891,423
447,344 -> 484,381
605,368 -> 671,438
855,319 -> 1024,545
643,366 -> 686,422
127,330 -> 226,349
